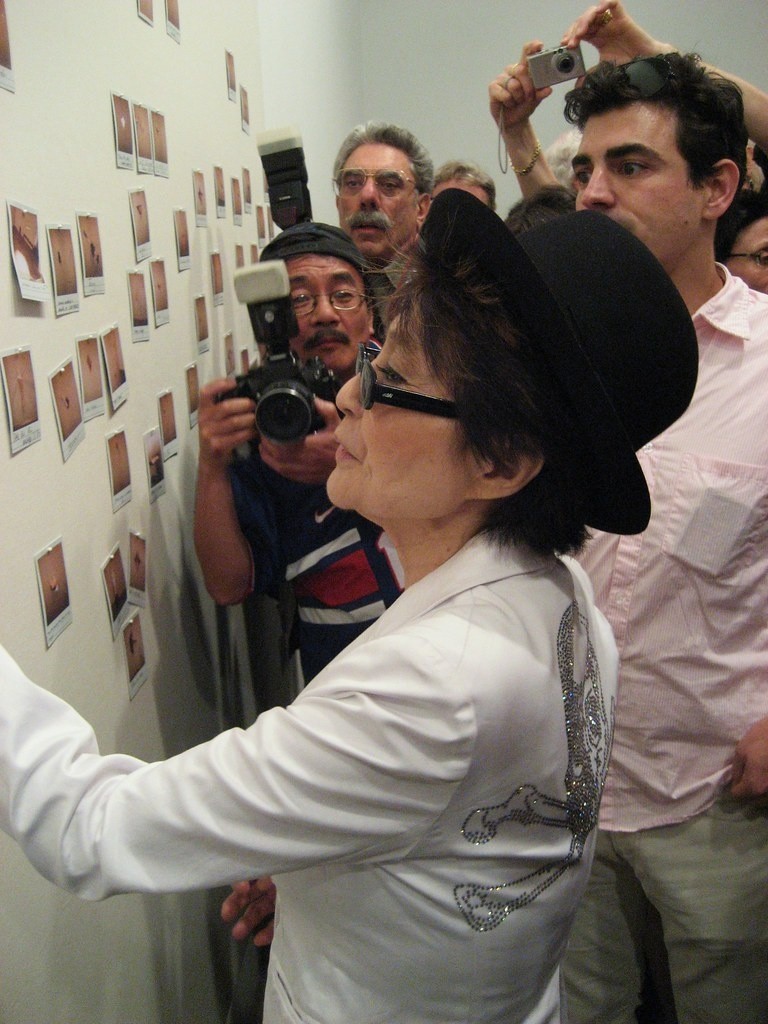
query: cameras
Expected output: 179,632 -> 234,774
526,44 -> 587,91
258,124 -> 313,232
214,259 -> 347,448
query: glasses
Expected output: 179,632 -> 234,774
356,341 -> 469,422
331,166 -> 419,197
728,249 -> 768,269
293,287 -> 371,315
580,56 -> 678,100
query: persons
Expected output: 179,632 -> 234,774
551,54 -> 768,1024
197,0 -> 768,686
0,190 -> 698,1024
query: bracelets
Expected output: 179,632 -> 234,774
513,141 -> 542,177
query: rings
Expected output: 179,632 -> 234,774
603,9 -> 612,23
511,63 -> 520,76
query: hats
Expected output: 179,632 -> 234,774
259,222 -> 385,340
713,188 -> 768,264
417,188 -> 698,536
